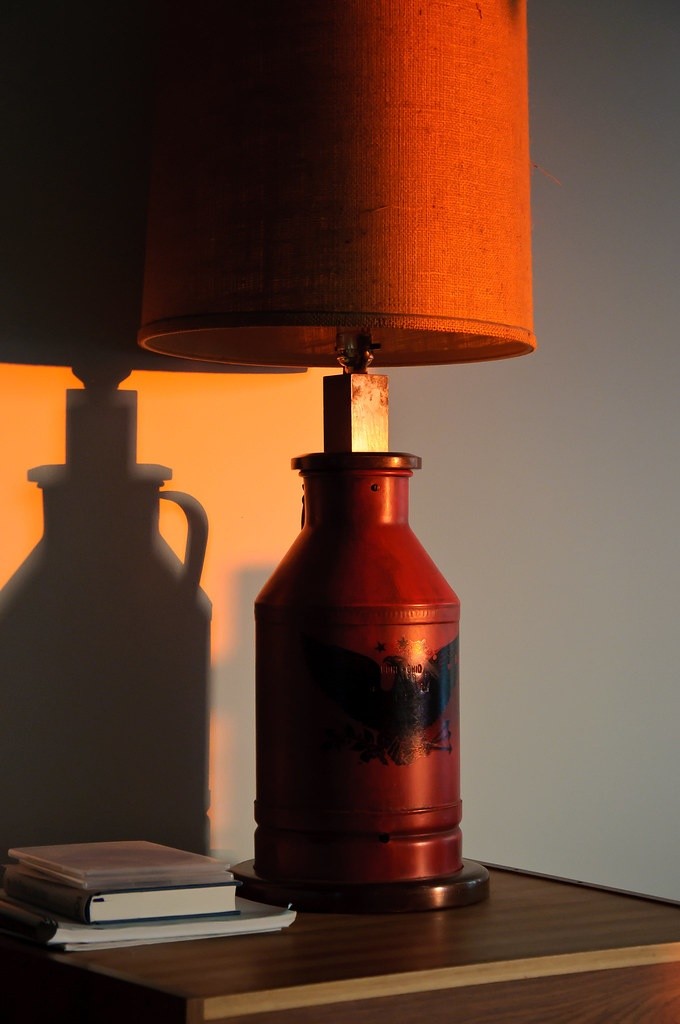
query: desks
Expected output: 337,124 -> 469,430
0,861 -> 680,1024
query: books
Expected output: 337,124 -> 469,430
0,841 -> 297,952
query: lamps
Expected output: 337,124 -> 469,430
135,0 -> 539,919
